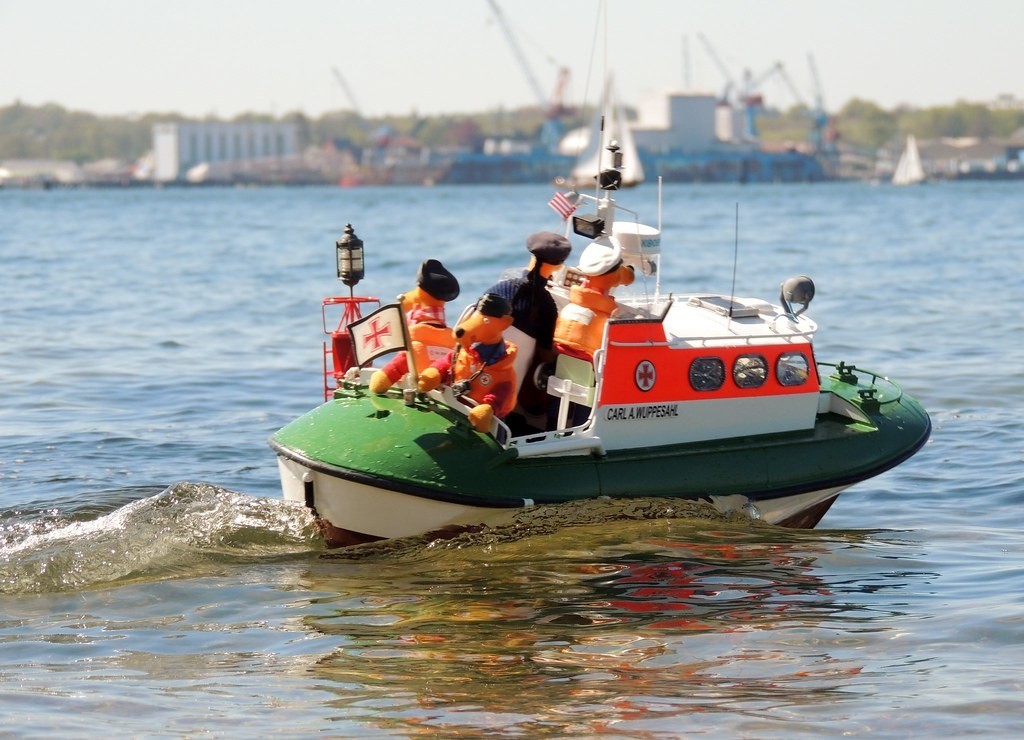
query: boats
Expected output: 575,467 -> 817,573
339,132 -> 456,187
266,116 -> 933,541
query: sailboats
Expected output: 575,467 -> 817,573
891,134 -> 926,185
565,72 -> 646,189
